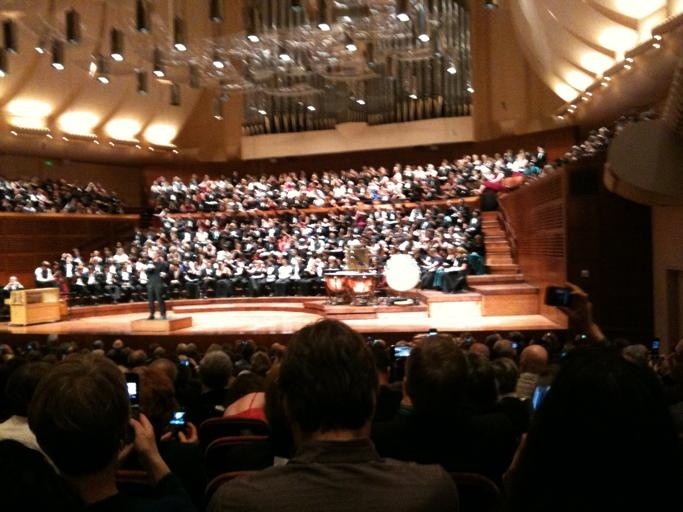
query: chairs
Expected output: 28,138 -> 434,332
114,415 -> 275,512
452,471 -> 504,511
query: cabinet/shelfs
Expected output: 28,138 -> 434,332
4,287 -> 60,326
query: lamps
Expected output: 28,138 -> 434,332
0,0 -> 476,120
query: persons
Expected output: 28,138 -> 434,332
144,250 -> 166,320
1,174 -> 125,213
131,145 -> 551,302
0,283 -> 683,511
52,226 -> 147,306
561,109 -> 659,162
2,275 -> 24,295
33,260 -> 59,288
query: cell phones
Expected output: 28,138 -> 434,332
123,372 -> 140,422
651,337 -> 662,367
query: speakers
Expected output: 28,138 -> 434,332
479,192 -> 498,212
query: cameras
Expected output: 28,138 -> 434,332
168,408 -> 187,425
543,283 -> 576,307
390,344 -> 416,358
